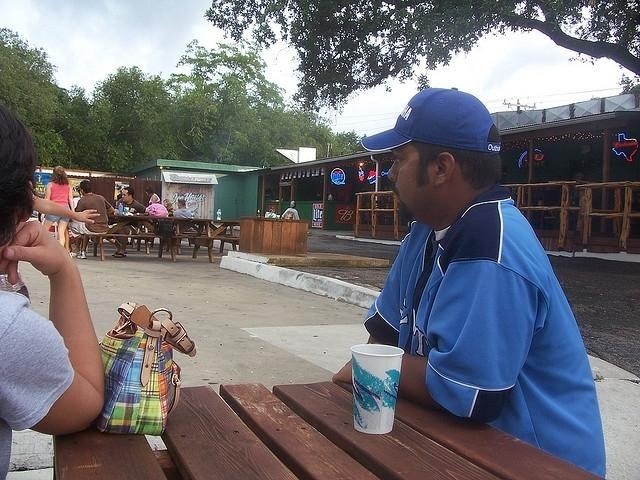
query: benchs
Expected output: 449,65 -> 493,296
192,235 -> 240,263
89,233 -> 155,260
161,236 -> 201,260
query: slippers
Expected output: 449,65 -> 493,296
112,251 -> 127,258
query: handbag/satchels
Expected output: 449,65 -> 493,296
96,301 -> 197,435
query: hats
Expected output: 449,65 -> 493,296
359,86 -> 502,156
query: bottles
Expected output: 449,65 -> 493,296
118,202 -> 124,214
216,208 -> 222,221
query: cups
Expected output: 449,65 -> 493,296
350,343 -> 405,434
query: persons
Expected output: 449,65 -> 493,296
0,103 -> 106,480
333,87 -> 606,475
32,165 -> 195,257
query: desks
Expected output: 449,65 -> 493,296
53,376 -> 599,476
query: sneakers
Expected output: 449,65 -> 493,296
76,251 -> 86,259
